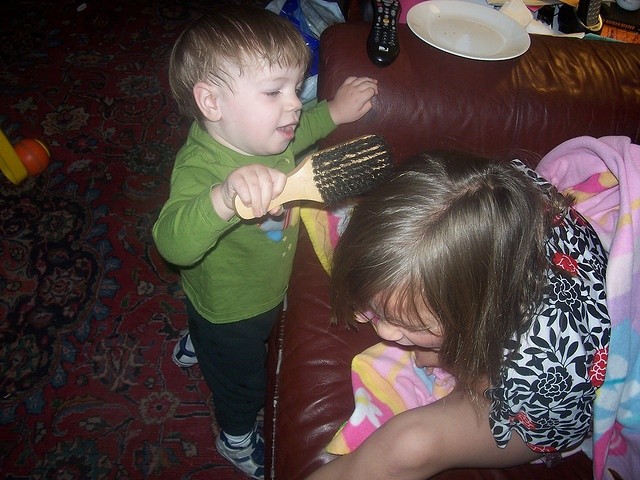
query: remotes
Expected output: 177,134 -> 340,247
367,0 -> 402,67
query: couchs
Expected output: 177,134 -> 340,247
264,21 -> 640,478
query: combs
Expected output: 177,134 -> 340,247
233,132 -> 394,220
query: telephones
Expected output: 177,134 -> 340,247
573,0 -> 604,35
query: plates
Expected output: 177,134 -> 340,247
405,0 -> 531,62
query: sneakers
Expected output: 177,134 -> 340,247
215,428 -> 266,480
172,330 -> 199,368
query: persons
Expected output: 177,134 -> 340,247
305,148 -> 610,480
149,5 -> 379,479
